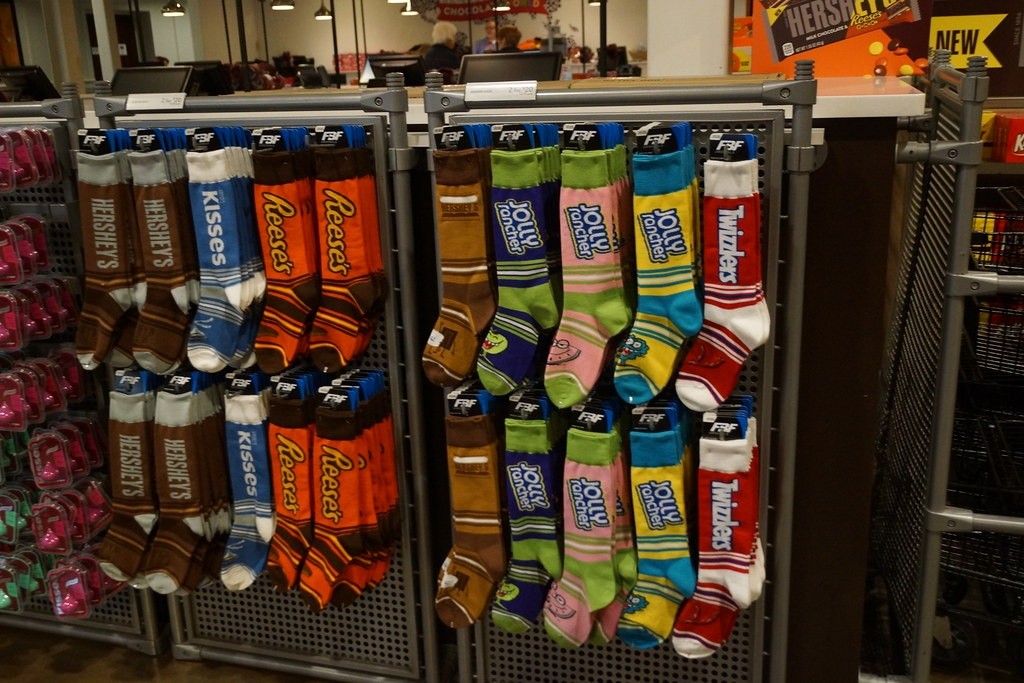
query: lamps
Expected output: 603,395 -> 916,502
161,0 -> 185,17
314,0 -> 333,21
491,0 -> 511,11
270,0 -> 295,11
400,0 -> 421,16
588,0 -> 601,6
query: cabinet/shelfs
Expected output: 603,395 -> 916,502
0,48 -> 1024,683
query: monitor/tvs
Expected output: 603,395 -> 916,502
111,60 -> 235,96
0,65 -> 62,102
457,50 -> 563,85
368,55 -> 429,88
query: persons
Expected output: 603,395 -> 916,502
453,21 -> 497,63
424,21 -> 460,69
491,25 -> 523,54
